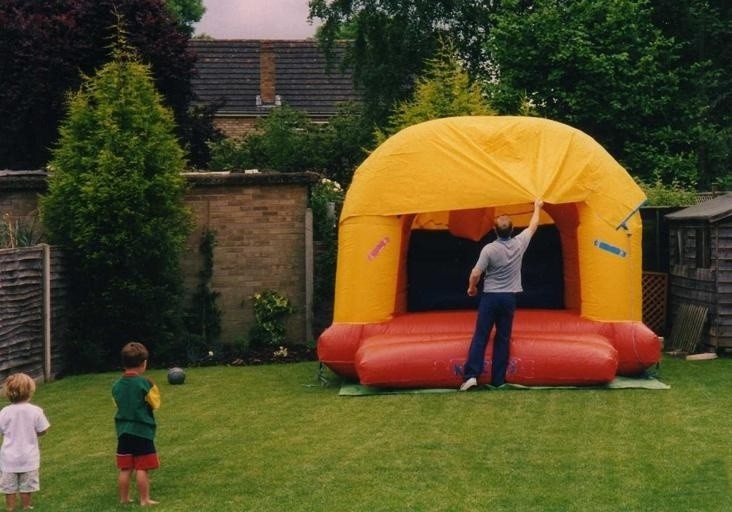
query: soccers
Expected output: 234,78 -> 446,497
168,368 -> 185,385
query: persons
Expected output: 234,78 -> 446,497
112,343 -> 161,506
459,197 -> 543,391
0,374 -> 51,512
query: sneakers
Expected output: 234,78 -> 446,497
459,377 -> 478,392
